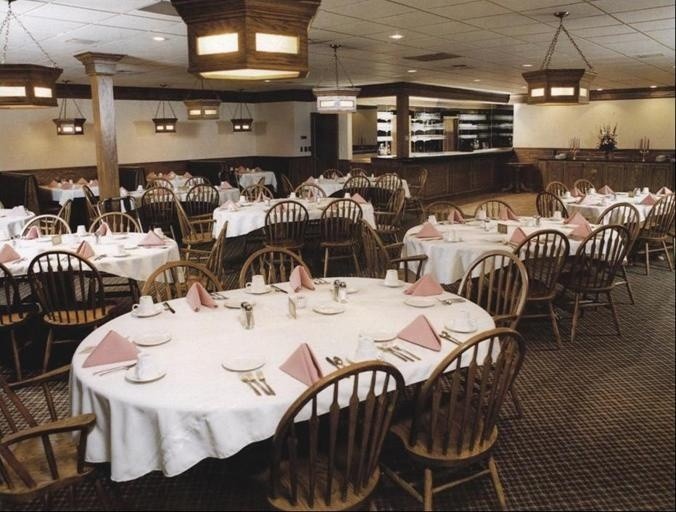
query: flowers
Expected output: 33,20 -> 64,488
596,121 -> 622,151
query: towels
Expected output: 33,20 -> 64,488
278,343 -> 323,386
394,314 -> 443,353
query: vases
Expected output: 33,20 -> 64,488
604,153 -> 614,159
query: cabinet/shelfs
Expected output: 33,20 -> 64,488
538,157 -> 675,196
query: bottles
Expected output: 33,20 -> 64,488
239,302 -> 254,329
295,293 -> 307,311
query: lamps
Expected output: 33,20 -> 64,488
311,43 -> 360,114
520,8 -> 599,105
0,0 -> 64,112
230,88 -> 253,133
52,78 -> 86,136
150,82 -> 179,135
180,75 -> 220,121
170,0 -> 320,82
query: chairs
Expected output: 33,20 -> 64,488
257,359 -> 407,510
380,329 -> 527,512
457,247 -> 530,422
0,168 -> 674,382
0,363 -> 111,511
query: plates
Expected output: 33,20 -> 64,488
125,362 -> 163,383
137,306 -> 164,316
223,350 -> 266,372
244,288 -> 271,294
223,300 -> 255,308
132,333 -> 170,348
314,280 -> 481,346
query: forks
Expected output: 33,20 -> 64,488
238,370 -> 280,396
324,356 -> 340,370
376,344 -> 420,363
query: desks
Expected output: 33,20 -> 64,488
501,161 -> 535,194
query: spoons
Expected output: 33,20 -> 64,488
334,355 -> 348,369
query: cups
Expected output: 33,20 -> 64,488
59,166 -> 375,205
564,186 -> 649,204
384,269 -> 399,283
0,225 -> 164,263
419,209 -> 599,243
246,274 -> 266,292
131,295 -> 152,315
